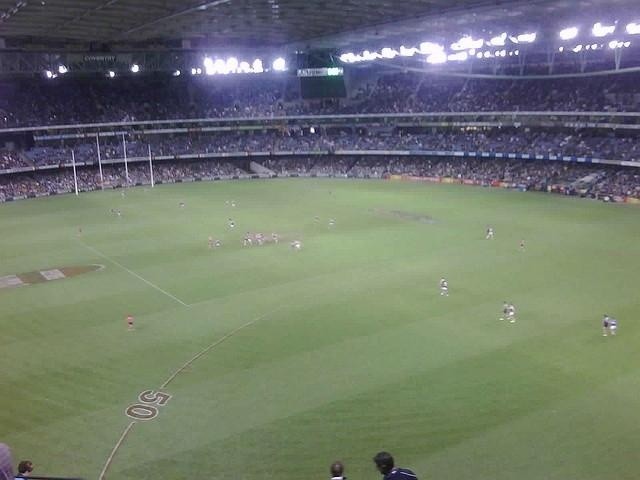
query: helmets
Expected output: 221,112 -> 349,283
373,452 -> 393,467
330,462 -> 343,475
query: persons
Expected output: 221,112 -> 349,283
601,312 -> 616,337
439,276 -> 449,296
500,301 -> 508,320
204,201 -> 339,255
373,452 -> 420,480
485,224 -> 496,240
330,461 -> 347,480
12,460 -> 34,480
102,204 -> 124,220
0,68 -> 640,207
507,303 -> 517,323
127,314 -> 136,336
